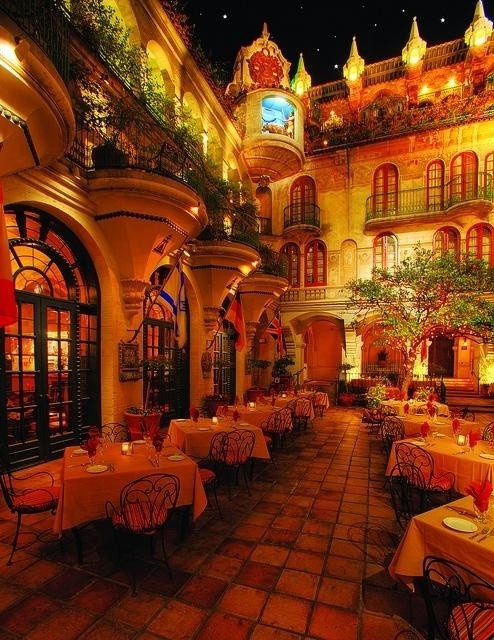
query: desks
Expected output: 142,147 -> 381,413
218,403 -> 292,457
166,416 -> 270,473
52,439 -> 209,540
256,392 -> 328,433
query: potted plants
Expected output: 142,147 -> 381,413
204,361 -> 232,416
123,356 -> 172,441
247,360 -> 271,404
337,364 -> 358,407
81,77 -> 140,168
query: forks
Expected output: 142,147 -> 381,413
469,527 -> 488,540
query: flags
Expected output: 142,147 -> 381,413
260,309 -> 288,359
224,284 -> 247,352
153,247 -> 187,340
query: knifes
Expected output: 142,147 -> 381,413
476,528 -> 494,543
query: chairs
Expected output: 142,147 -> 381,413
105,473 -> 181,596
218,428 -> 259,501
194,430 -> 229,520
1,443 -> 61,565
97,422 -> 129,441
361,398 -> 494,639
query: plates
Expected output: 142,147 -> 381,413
238,422 -> 249,427
394,412 -> 494,462
175,419 -> 187,421
86,464 -> 106,473
442,517 -> 477,533
72,449 -> 88,455
167,455 -> 184,461
198,427 -> 209,431
132,440 -> 146,444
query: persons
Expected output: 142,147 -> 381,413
282,112 -> 295,137
